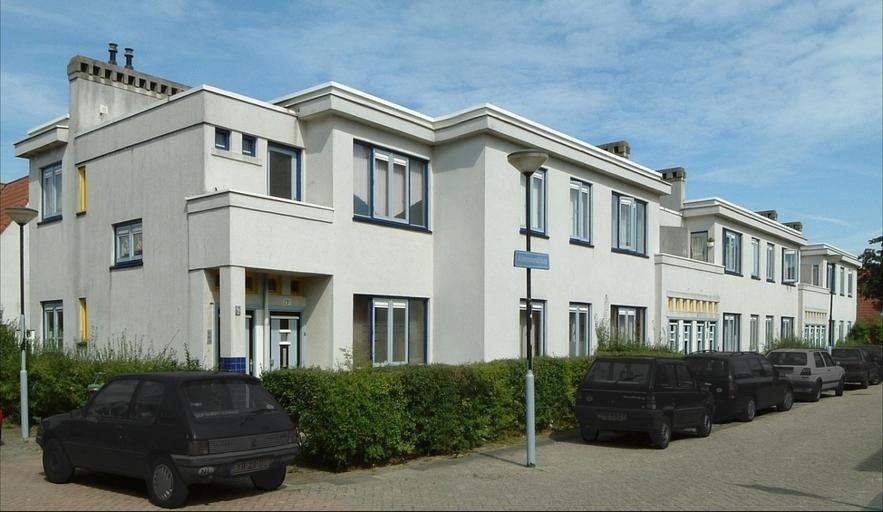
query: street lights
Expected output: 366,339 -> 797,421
825,254 -> 843,355
3,206 -> 38,438
506,150 -> 549,468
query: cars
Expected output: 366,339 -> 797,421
35,371 -> 298,509
